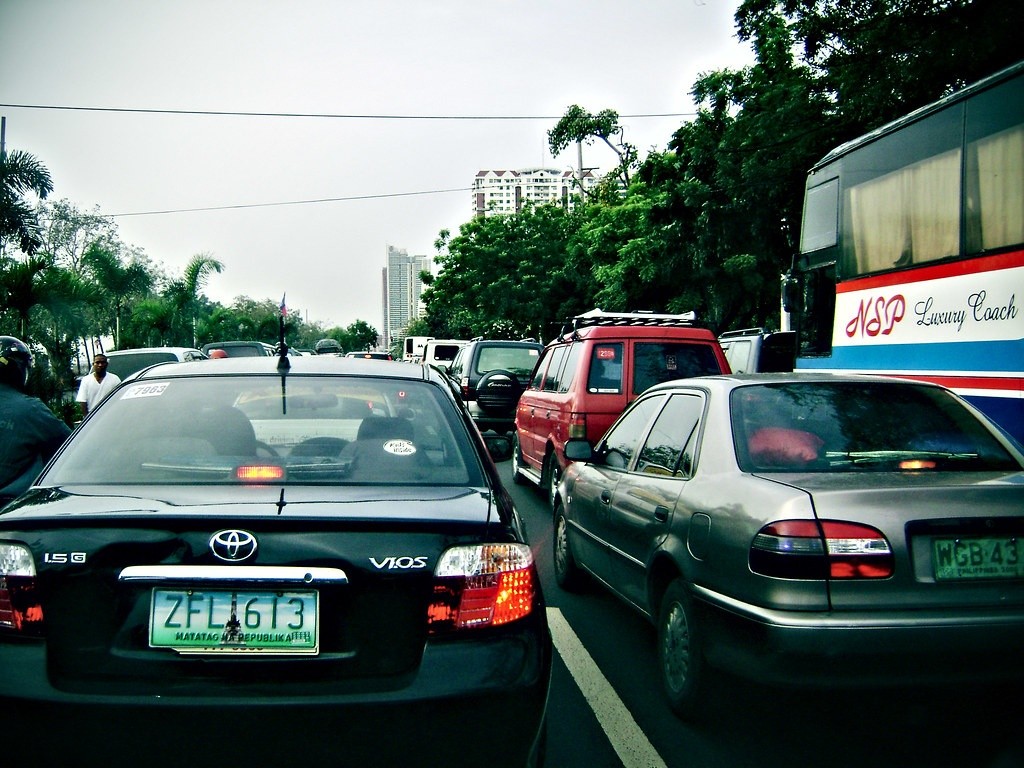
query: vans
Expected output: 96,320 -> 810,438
200,337 -> 470,374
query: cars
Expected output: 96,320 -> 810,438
552,369 -> 1024,717
228,385 -> 417,447
88,348 -> 208,385
0,358 -> 554,768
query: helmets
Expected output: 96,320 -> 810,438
0,336 -> 35,386
211,350 -> 228,358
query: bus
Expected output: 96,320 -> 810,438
779,56 -> 1024,457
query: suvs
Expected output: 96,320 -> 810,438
437,336 -> 545,446
716,326 -> 795,375
509,308 -> 734,513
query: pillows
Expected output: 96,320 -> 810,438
750,428 -> 825,465
905,433 -> 1012,462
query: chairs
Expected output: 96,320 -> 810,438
358,417 -> 414,439
200,406 -> 258,457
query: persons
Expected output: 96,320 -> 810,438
210,349 -> 228,360
0,335 -> 73,510
74,354 -> 122,419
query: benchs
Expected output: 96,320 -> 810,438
131,437 -> 436,469
